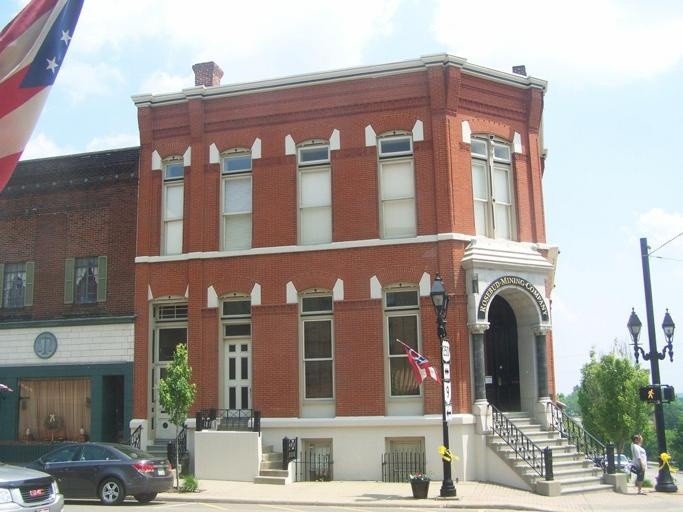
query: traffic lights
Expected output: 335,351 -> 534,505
638,385 -> 659,401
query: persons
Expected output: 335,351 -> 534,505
631,435 -> 647,495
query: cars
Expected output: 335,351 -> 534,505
23,441 -> 174,505
584,452 -> 632,484
0,461 -> 65,512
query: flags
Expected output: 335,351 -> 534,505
396,339 -> 442,385
0,0 -> 84,193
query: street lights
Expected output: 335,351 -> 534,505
624,237 -> 679,494
429,272 -> 458,500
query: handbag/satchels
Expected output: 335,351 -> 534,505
630,465 -> 641,474
